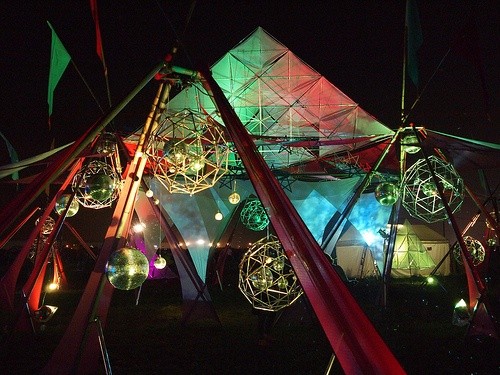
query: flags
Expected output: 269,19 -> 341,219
90,0 -> 105,61
1,133 -> 21,180
406,0 -> 423,92
47,29 -> 72,127
48,113 -> 56,151
